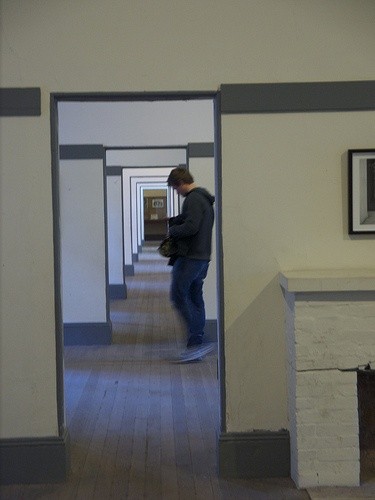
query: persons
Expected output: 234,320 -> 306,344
166,167 -> 215,363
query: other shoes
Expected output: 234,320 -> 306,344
169,340 -> 215,367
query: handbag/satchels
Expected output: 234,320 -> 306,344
156,214 -> 186,266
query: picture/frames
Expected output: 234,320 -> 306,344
347,148 -> 375,235
